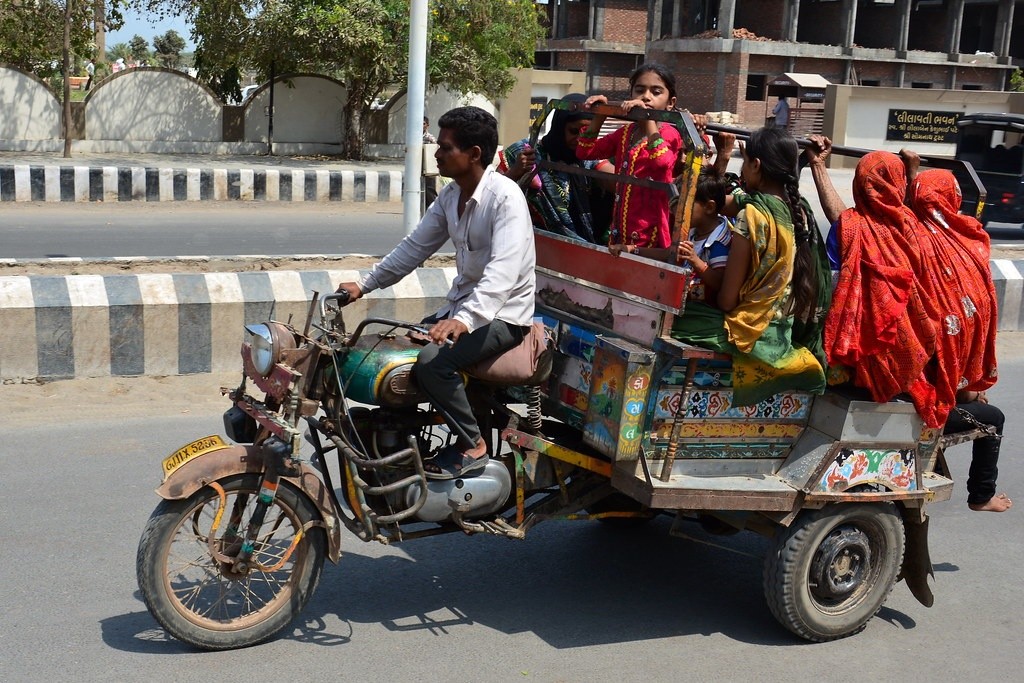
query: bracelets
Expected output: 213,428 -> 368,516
976,390 -> 981,401
699,262 -> 709,274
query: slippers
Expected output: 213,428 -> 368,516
423,443 -> 489,479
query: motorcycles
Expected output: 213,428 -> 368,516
957,111 -> 1024,225
135,96 -> 1003,654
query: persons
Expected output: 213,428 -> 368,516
773,94 -> 790,128
423,116 -> 439,210
497,62 -> 1014,512
335,107 -> 537,480
